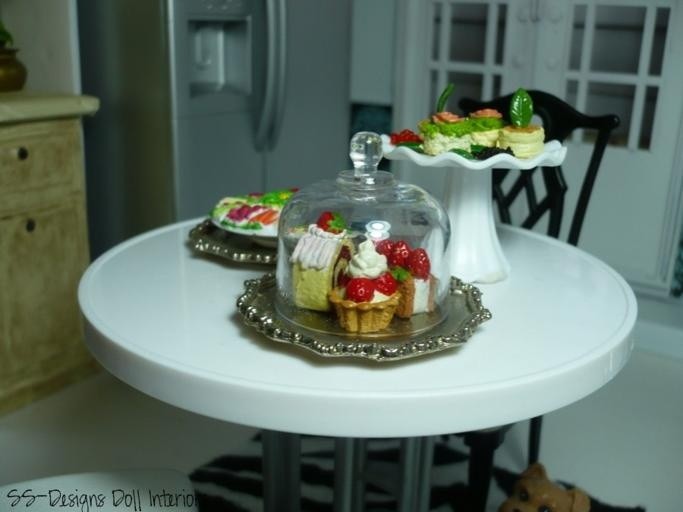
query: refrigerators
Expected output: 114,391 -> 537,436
79,1 -> 353,264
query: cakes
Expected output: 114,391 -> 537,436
419,82 -> 545,160
288,211 -> 438,335
212,186 -> 299,231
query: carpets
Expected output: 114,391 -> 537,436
181,431 -> 618,512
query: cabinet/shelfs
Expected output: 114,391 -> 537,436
0,116 -> 96,411
402,0 -> 683,286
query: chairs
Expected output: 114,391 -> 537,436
462,90 -> 621,508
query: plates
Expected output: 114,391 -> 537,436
211,198 -> 285,243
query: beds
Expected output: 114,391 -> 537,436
76,188 -> 640,512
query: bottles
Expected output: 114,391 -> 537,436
0,47 -> 28,94
